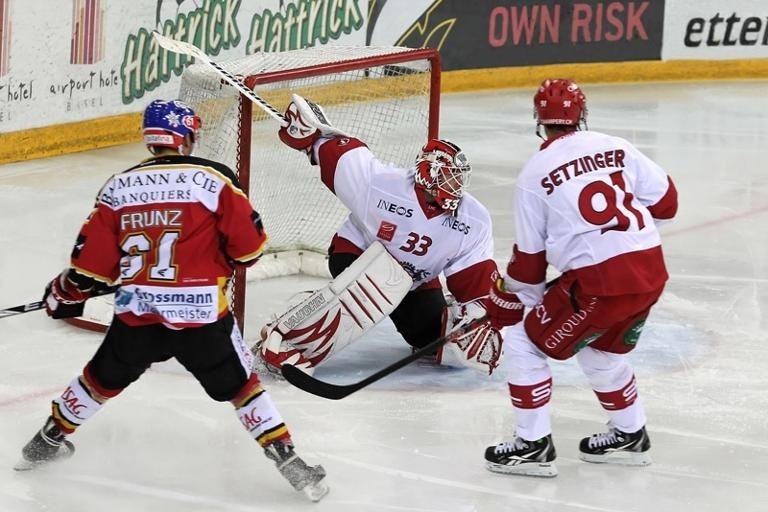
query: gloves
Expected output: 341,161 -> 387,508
43,269 -> 95,319
487,277 -> 524,326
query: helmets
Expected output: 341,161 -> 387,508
533,78 -> 586,126
415,139 -> 472,211
143,99 -> 202,150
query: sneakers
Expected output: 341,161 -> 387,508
485,434 -> 556,466
579,427 -> 650,455
277,450 -> 326,491
22,428 -> 75,462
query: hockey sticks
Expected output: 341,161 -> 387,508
282,279 -> 559,398
152,32 -> 289,128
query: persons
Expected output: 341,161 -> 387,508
21,99 -> 325,492
278,92 -> 493,365
484,78 -> 678,478
